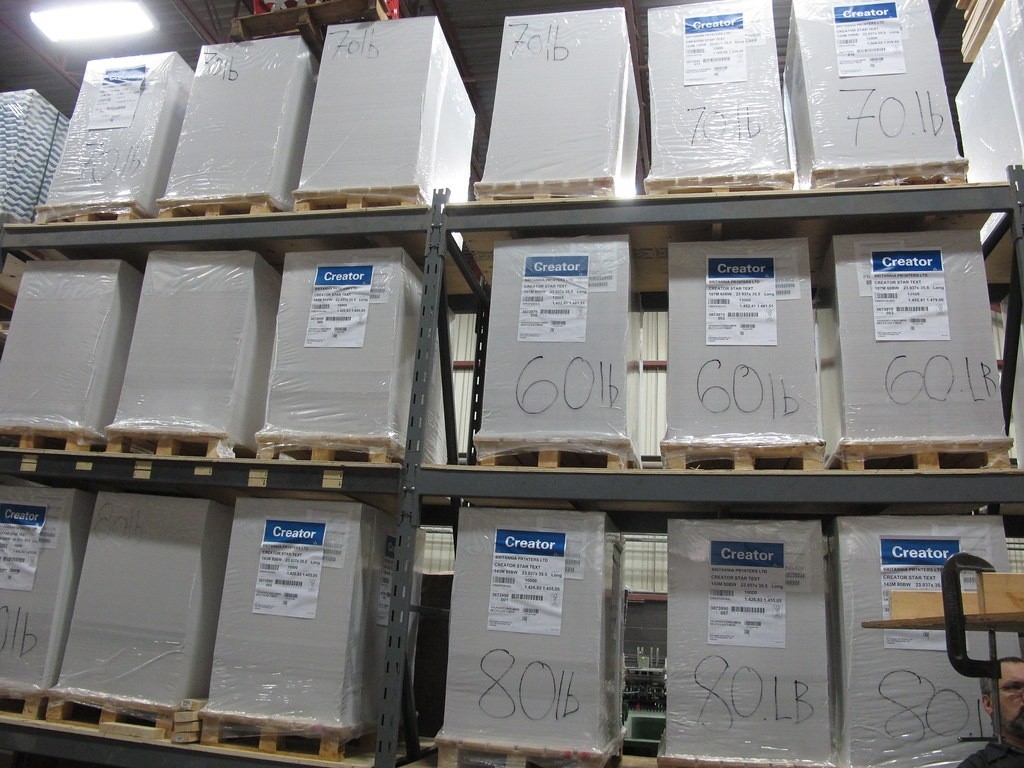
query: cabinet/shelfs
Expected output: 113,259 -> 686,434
0,162 -> 1023,767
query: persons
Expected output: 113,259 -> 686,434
955,657 -> 1023,768
